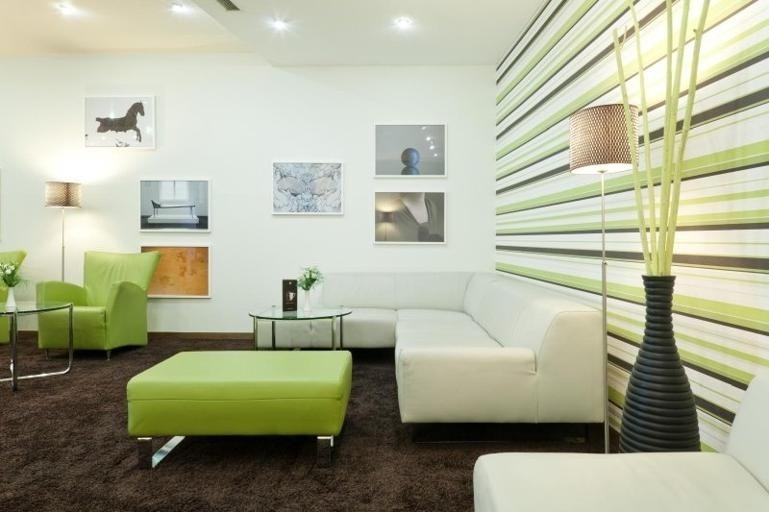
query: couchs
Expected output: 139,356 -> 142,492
396,269 -> 609,443
251,268 -> 471,361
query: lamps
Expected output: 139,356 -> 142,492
565,101 -> 639,454
43,178 -> 83,282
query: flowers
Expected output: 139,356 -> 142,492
0,259 -> 20,287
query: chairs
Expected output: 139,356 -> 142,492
0,248 -> 29,347
35,248 -> 163,363
469,366 -> 768,511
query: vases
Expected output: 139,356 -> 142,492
615,273 -> 704,454
4,286 -> 17,309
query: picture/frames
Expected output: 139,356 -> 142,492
367,189 -> 447,246
267,159 -> 348,218
78,91 -> 159,152
136,176 -> 213,234
137,242 -> 215,300
366,119 -> 451,181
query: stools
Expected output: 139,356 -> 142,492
126,349 -> 356,472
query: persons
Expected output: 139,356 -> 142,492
381,193 -> 441,243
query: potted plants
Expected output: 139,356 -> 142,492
296,265 -> 324,314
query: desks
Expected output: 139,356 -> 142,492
2,301 -> 77,393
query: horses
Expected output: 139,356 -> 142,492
85,102 -> 145,142
